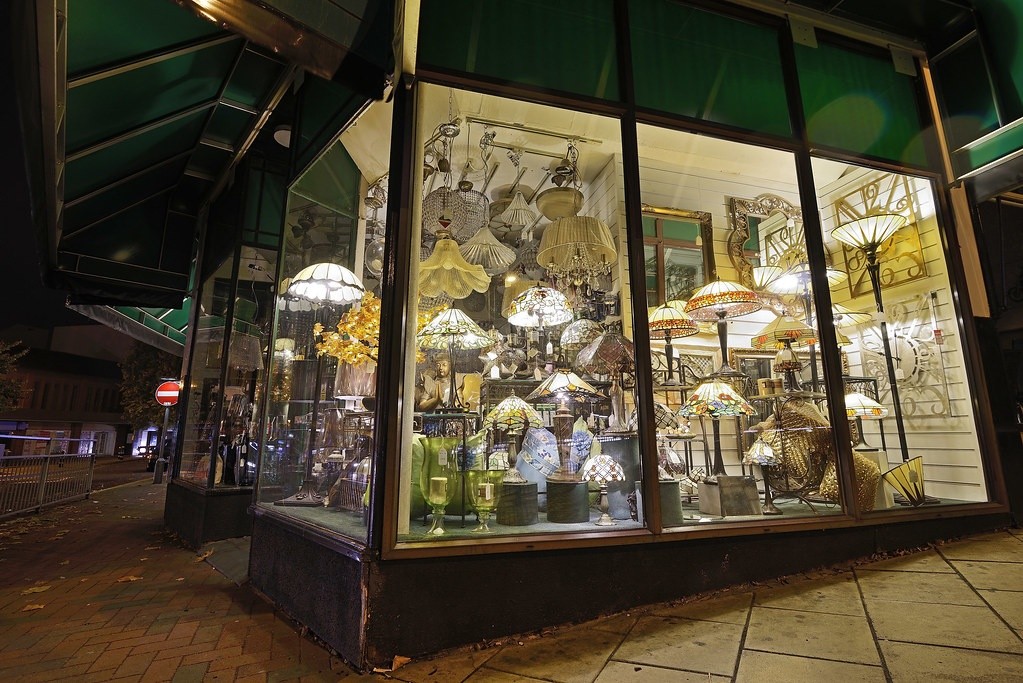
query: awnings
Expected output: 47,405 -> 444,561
0,0 -> 376,309
49,292 -> 188,359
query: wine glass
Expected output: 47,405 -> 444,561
418,436 -> 459,535
458,468 -> 508,533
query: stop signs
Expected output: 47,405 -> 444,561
155,381 -> 181,407
